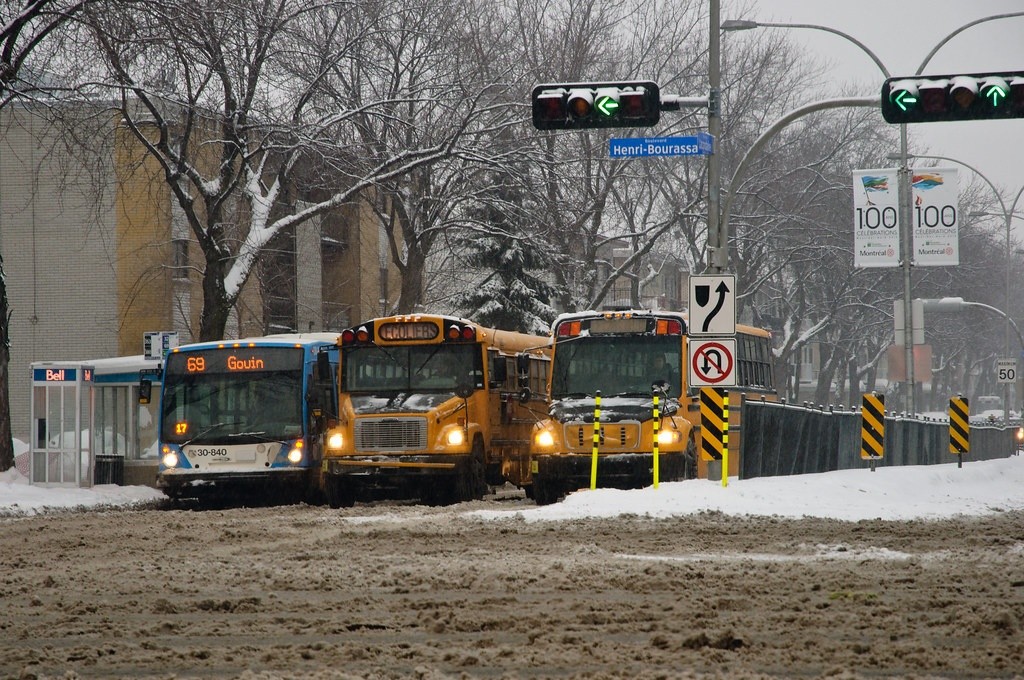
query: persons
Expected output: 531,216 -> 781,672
647,353 -> 668,376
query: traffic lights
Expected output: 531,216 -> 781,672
882,71 -> 1024,123
531,84 -> 662,131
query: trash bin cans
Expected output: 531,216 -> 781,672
93,454 -> 124,487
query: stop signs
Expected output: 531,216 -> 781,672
506,395 -> 513,418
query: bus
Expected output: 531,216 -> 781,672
312,311 -> 600,510
978,396 -> 1002,416
136,330 -> 414,507
516,311 -> 780,504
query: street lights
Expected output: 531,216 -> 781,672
886,153 -> 1011,425
720,11 -> 911,416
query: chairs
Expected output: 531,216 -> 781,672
568,363 -> 680,393
356,358 -> 469,387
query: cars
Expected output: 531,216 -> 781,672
997,419 -> 1024,443
